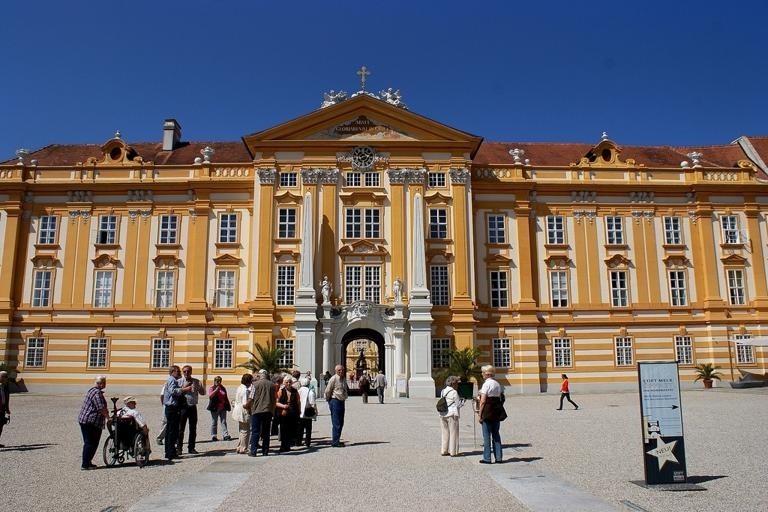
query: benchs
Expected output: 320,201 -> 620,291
729,373 -> 768,389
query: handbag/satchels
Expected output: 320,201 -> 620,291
305,407 -> 316,417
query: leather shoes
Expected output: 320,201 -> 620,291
157,438 -> 198,459
479,460 -> 491,463
333,442 -> 345,446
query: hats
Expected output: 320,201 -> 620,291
125,397 -> 136,404
481,365 -> 494,375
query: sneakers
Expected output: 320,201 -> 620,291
81,464 -> 97,470
212,436 -> 231,441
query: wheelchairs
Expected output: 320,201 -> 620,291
102,416 -> 152,467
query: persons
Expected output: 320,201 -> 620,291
438,376 -> 465,456
394,276 -> 402,302
77,376 -> 110,470
117,396 -> 152,455
0,371 -> 11,447
319,275 -> 333,304
478,365 -> 508,464
156,364 -> 387,459
557,374 -> 579,410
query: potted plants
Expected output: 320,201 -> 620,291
439,345 -> 485,399
694,363 -> 721,388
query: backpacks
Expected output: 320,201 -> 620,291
437,390 -> 454,412
360,376 -> 370,391
